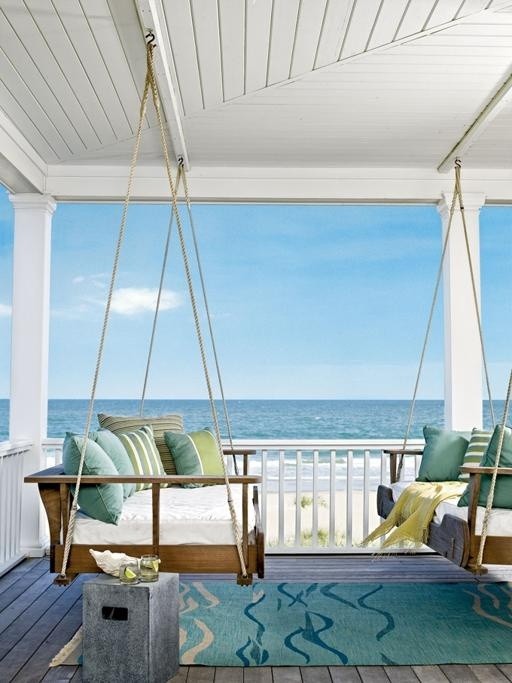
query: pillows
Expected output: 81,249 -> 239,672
415,423 -> 512,511
61,411 -> 227,527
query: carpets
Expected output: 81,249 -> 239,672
46,576 -> 512,673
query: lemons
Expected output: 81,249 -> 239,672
152,560 -> 159,572
124,567 -> 136,578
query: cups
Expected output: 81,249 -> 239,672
139,554 -> 160,582
118,557 -> 140,585
